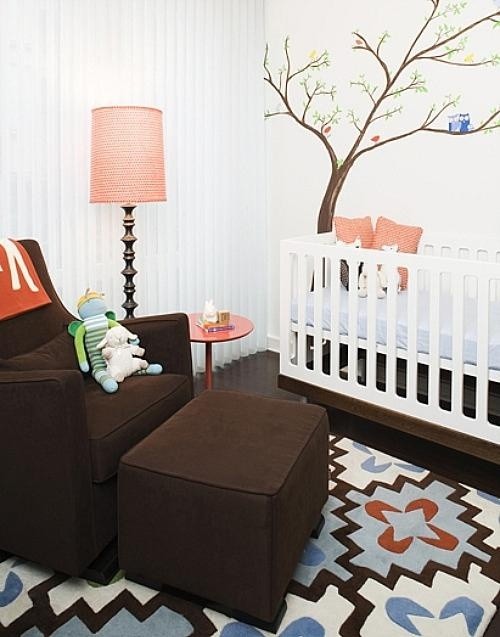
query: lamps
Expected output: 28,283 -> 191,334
88,104 -> 168,318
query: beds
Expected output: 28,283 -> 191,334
278,231 -> 500,467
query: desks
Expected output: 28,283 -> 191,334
187,312 -> 254,389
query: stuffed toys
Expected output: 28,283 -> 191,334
203,298 -> 219,323
335,238 -> 402,297
95,324 -> 150,383
68,286 -> 163,393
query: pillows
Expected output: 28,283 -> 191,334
371,215 -> 423,289
333,215 -> 374,249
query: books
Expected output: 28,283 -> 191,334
196,317 -> 236,333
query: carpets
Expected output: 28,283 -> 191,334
0,432 -> 500,637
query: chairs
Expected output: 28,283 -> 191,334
0,237 -> 196,577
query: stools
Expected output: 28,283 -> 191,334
116,390 -> 329,635
